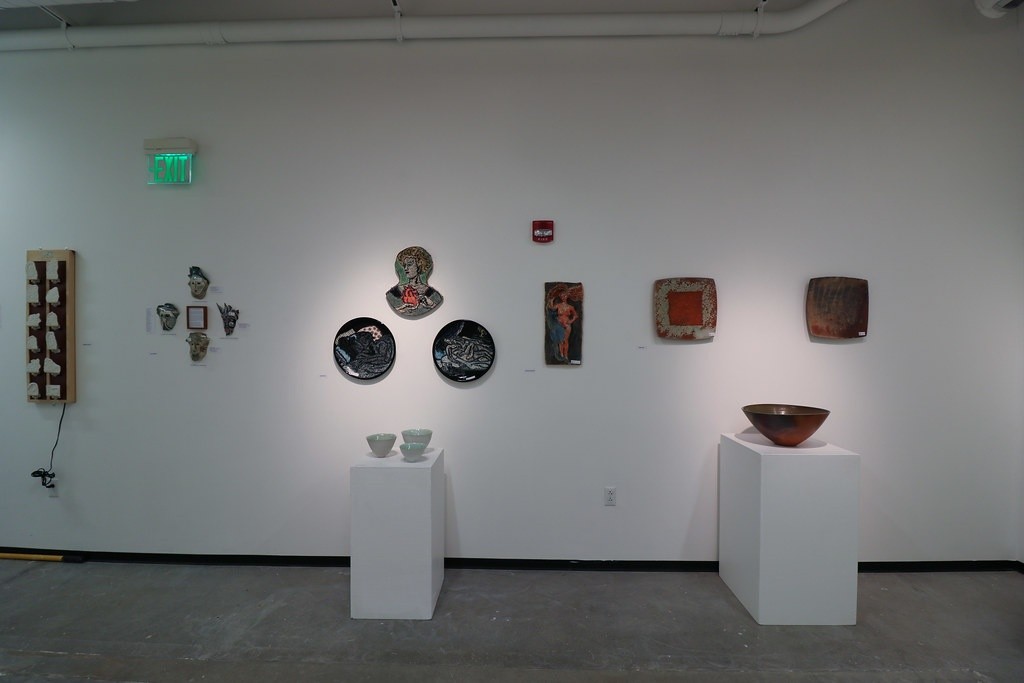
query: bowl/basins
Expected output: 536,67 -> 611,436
400,443 -> 425,462
743,403 -> 830,447
401,429 -> 433,449
366,433 -> 397,457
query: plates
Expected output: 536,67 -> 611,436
333,317 -> 396,380
431,320 -> 495,383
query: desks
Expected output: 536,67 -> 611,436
719,432 -> 862,626
349,448 -> 446,620
